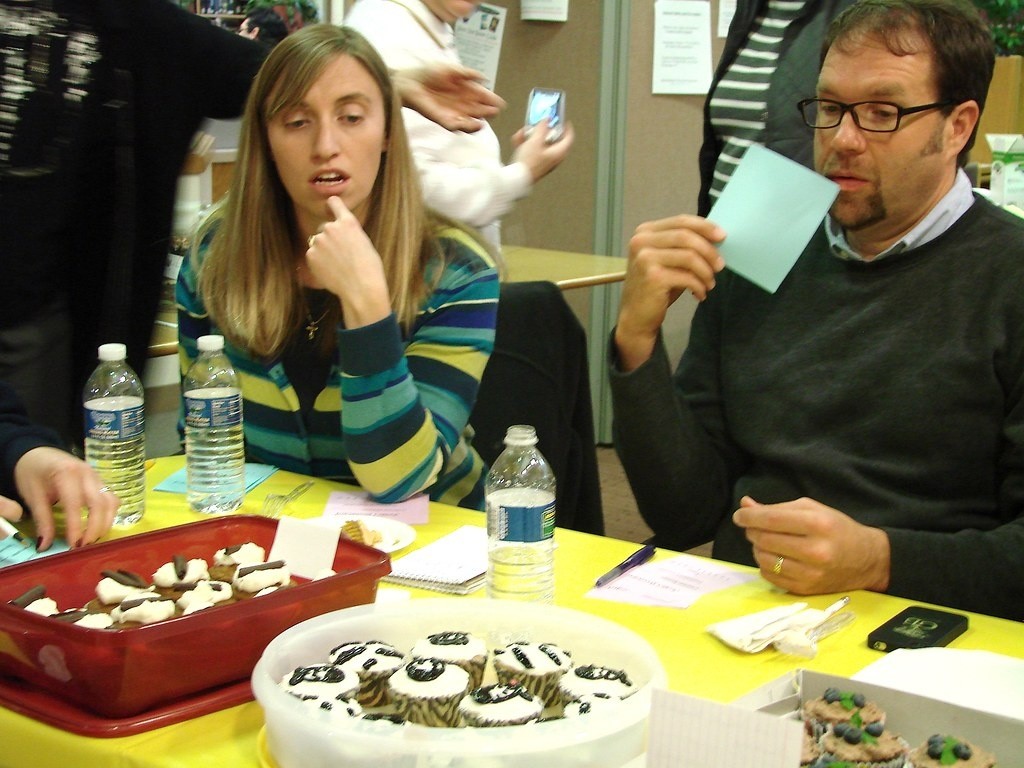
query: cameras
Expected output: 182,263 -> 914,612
524,87 -> 566,144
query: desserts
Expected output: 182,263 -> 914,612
8,542 -> 300,630
800,688 -> 997,768
343,519 -> 381,546
278,631 -> 639,728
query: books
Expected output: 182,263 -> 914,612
377,523 -> 560,595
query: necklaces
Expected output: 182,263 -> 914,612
295,267 -> 335,340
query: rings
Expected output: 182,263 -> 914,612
100,486 -> 110,493
772,556 -> 784,574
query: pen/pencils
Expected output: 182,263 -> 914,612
824,596 -> 850,615
593,544 -> 657,587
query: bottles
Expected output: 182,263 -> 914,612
485,425 -> 555,603
82,343 -> 146,525
182,335 -> 245,516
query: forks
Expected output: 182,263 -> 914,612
260,481 -> 315,520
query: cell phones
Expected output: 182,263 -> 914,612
868,606 -> 969,653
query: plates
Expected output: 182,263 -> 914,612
320,514 -> 416,554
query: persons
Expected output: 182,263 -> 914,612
0,0 -> 509,447
698,0 -> 859,218
0,414 -> 120,551
238,10 -> 288,48
342,0 -> 576,282
172,21 -> 500,513
605,0 -> 1024,619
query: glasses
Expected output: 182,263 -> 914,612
797,95 -> 961,133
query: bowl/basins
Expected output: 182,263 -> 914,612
1,513 -> 392,742
250,597 -> 663,768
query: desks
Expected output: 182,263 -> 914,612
0,454 -> 1024,768
151,244 -> 632,363
